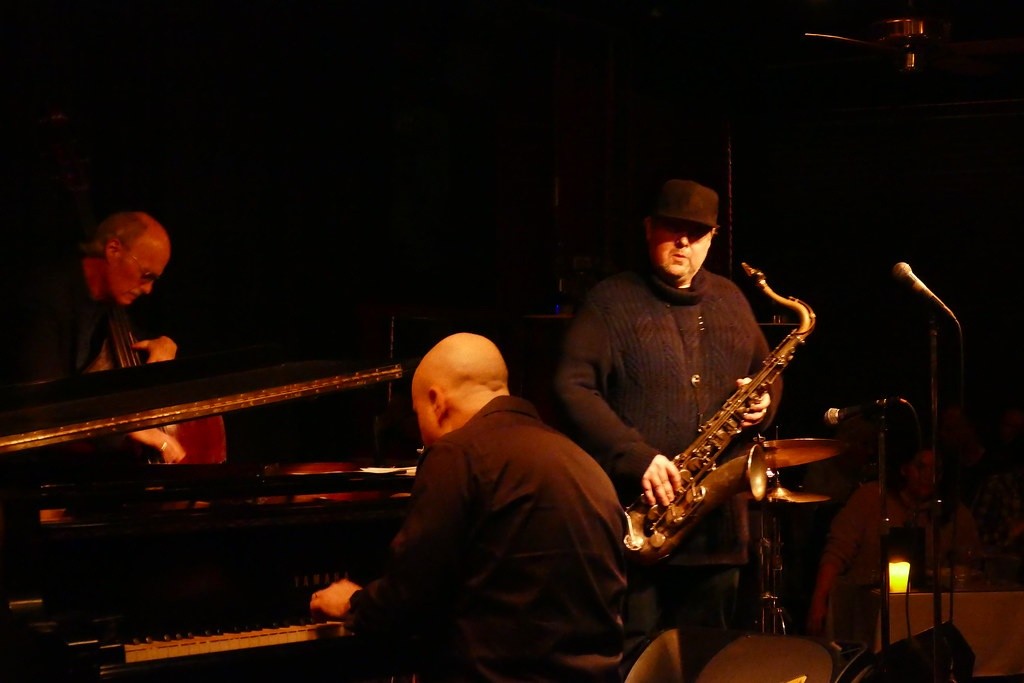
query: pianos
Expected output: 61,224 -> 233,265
0,345 -> 420,683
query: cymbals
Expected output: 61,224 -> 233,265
524,311 -> 577,319
739,485 -> 833,505
730,437 -> 850,470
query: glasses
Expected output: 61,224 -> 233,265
124,250 -> 161,284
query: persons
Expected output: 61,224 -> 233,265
555,178 -> 786,683
309,332 -> 627,683
0,210 -> 186,476
811,444 -> 992,683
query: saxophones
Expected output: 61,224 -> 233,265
622,260 -> 818,567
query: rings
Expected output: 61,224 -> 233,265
160,442 -> 168,451
313,591 -> 317,598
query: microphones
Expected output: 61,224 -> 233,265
824,395 -> 903,426
891,262 -> 956,320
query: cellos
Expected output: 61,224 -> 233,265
36,105 -> 230,467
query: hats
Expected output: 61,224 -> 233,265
656,180 -> 721,228
